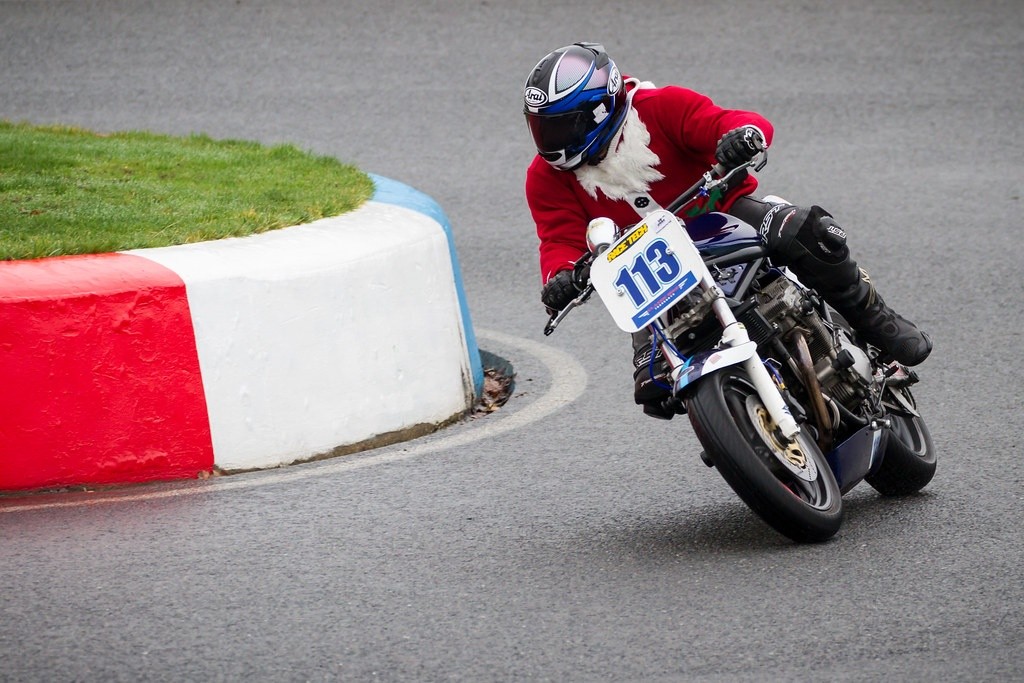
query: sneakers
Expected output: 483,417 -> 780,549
855,292 -> 933,364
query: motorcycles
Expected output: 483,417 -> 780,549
549,127 -> 935,543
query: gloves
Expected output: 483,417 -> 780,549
715,126 -> 763,168
540,269 -> 579,310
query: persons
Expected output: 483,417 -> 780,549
522,42 -> 934,419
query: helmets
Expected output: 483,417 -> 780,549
522,42 -> 627,172
